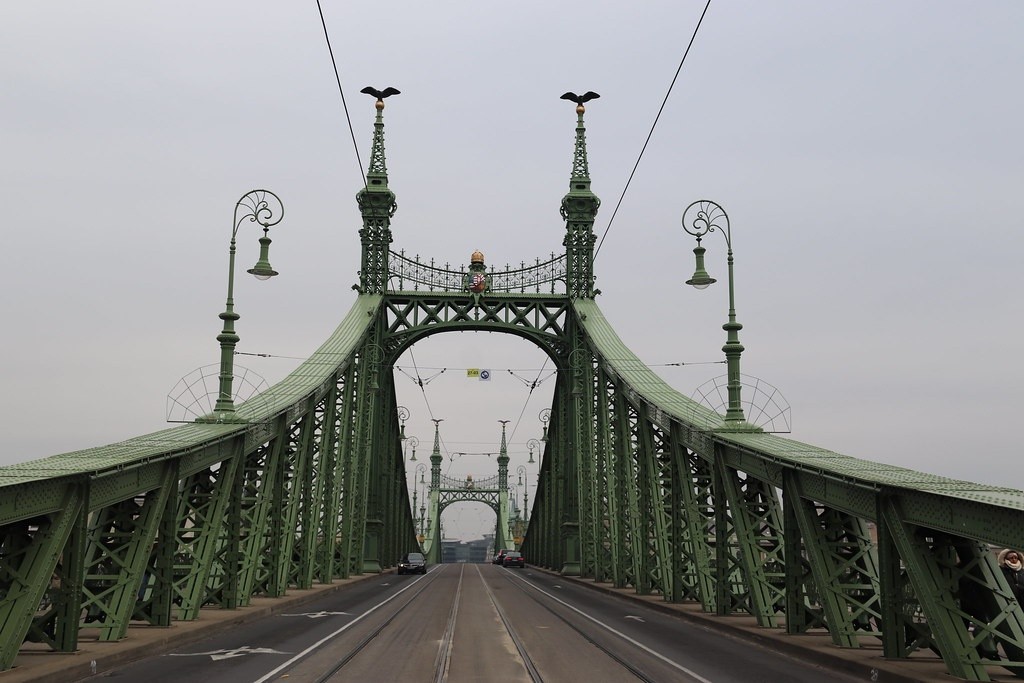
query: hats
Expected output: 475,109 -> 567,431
1007,552 -> 1018,558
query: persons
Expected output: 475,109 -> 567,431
996,548 -> 1024,614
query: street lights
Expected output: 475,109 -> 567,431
412,462 -> 427,530
404,436 -> 419,465
419,486 -> 433,554
214,189 -> 285,412
538,408 -> 559,450
526,438 -> 541,480
506,482 -> 520,550
389,406 -> 410,445
516,465 -> 529,532
681,199 -> 747,421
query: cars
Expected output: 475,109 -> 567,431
502,551 -> 526,569
492,548 -> 515,566
396,552 -> 428,575
